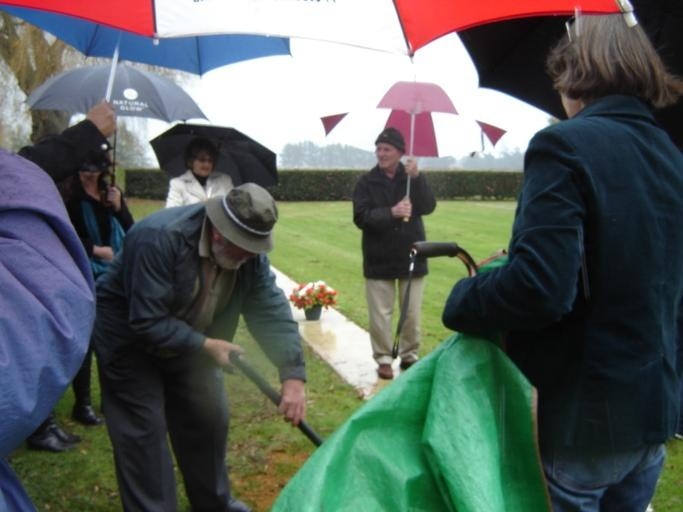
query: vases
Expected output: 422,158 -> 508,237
305,305 -> 321,320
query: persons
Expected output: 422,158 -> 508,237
441,15 -> 683,511
0,148 -> 96,512
352,127 -> 436,378
92,182 -> 307,512
61,157 -> 139,426
17,100 -> 116,454
164,138 -> 235,208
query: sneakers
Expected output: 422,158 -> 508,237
400,358 -> 419,370
376,362 -> 394,378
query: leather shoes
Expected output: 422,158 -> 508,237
27,424 -> 75,451
73,402 -> 104,425
45,422 -> 82,444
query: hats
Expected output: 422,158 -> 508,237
375,127 -> 406,155
198,183 -> 280,257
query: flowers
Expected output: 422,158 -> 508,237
290,280 -> 336,308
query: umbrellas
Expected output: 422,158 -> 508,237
319,79 -> 510,223
149,123 -> 280,192
0,0 -> 293,104
22,62 -> 211,186
393,0 -> 683,152
